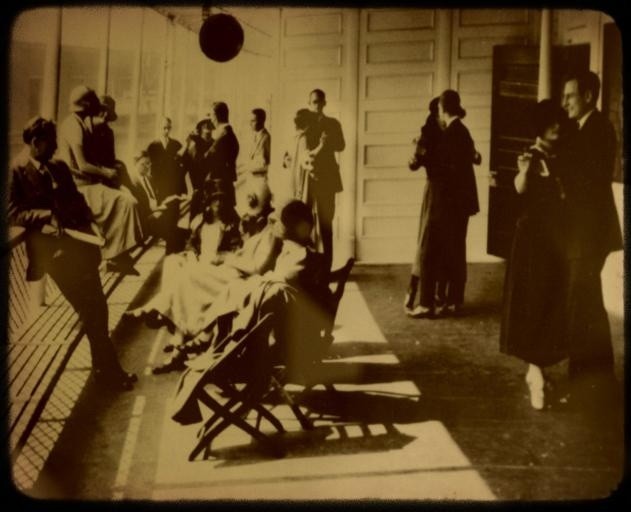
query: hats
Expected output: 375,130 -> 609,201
195,116 -> 217,132
68,85 -> 100,112
101,95 -> 117,121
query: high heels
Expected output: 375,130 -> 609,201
147,313 -> 176,334
121,309 -> 156,325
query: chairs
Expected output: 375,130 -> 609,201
261,257 -> 356,401
185,312 -> 285,460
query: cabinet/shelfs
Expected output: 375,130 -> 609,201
487,44 -> 590,260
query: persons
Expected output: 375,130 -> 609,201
144,117 -> 182,205
534,72 -> 624,379
185,191 -> 243,265
123,209 -> 287,338
498,99 -> 582,411
127,152 -> 183,250
161,213 -> 267,291
281,89 -> 346,271
6,115 -> 138,391
286,109 -> 328,249
203,100 -> 239,225
180,119 -> 215,235
86,94 -> 157,249
48,86 -> 141,277
246,108 -> 271,184
152,200 -> 330,375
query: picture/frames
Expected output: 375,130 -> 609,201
601,22 -> 624,182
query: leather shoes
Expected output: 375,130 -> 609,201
406,305 -> 435,319
123,371 -> 137,382
152,358 -> 187,375
119,380 -> 133,391
176,333 -> 212,354
